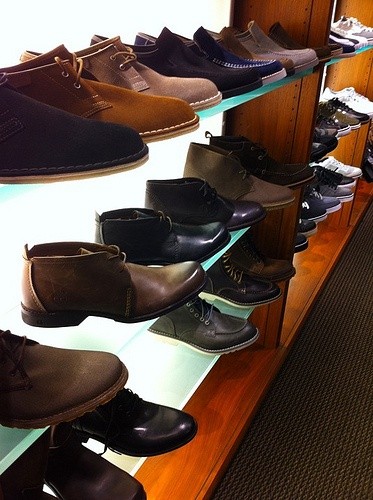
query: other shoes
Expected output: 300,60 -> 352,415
310,136 -> 338,153
294,234 -> 309,252
295,218 -> 318,236
0,455 -> 60,500
307,143 -> 327,160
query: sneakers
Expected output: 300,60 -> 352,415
316,102 -> 361,130
319,87 -> 373,117
309,166 -> 356,187
0,16 -> 373,184
302,185 -> 342,213
310,181 -> 355,202
309,156 -> 363,179
313,113 -> 352,137
327,99 -> 370,124
296,197 -> 328,222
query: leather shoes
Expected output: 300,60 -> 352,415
184,142 -> 296,210
94,208 -> 231,265
29,435 -> 148,500
229,238 -> 298,283
67,389 -> 198,457
21,241 -> 207,328
0,330 -> 130,430
146,177 -> 267,229
202,253 -> 283,308
207,131 -> 316,187
149,291 -> 260,355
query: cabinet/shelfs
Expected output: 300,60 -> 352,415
0,0 -> 373,500
